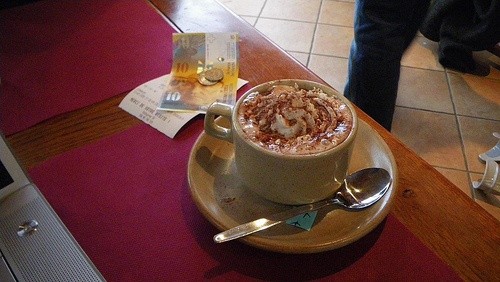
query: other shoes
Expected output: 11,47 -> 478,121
442,58 -> 491,77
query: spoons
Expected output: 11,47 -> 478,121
212,166 -> 392,243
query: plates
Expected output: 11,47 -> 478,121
187,78 -> 398,255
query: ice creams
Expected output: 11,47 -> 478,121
236,81 -> 352,155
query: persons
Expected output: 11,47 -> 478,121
342,0 -> 500,135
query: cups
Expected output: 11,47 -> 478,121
204,80 -> 359,204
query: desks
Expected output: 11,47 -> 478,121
0,0 -> 500,282
